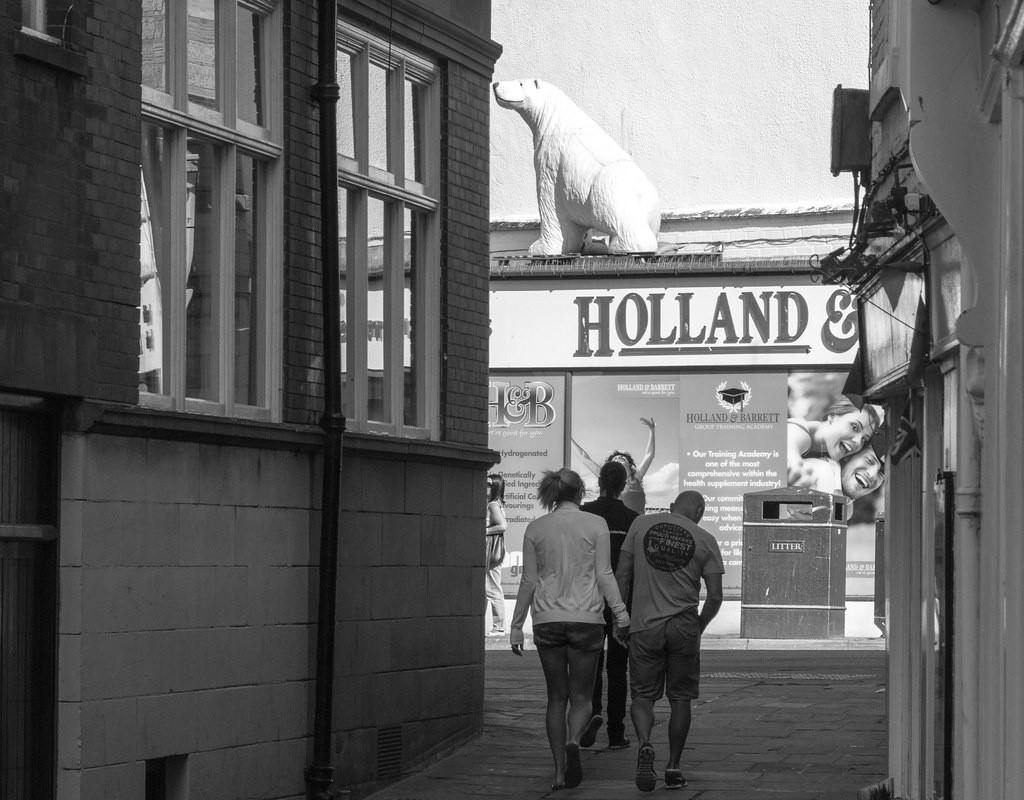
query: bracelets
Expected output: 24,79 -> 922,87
613,619 -> 617,623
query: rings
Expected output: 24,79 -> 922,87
622,634 -> 625,637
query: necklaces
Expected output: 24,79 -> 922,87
819,432 -> 826,458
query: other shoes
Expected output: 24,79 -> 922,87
564,742 -> 583,788
579,715 -> 604,747
665,771 -> 688,789
636,742 -> 657,792
609,738 -> 631,749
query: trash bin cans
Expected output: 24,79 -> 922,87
874,512 -> 886,638
740,486 -> 848,639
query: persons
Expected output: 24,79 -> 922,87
801,432 -> 885,524
569,417 -> 656,517
578,461 -> 641,748
612,490 -> 726,793
510,468 -> 632,789
484,474 -> 509,636
785,395 -> 880,493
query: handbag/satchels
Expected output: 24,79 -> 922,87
486,503 -> 505,570
